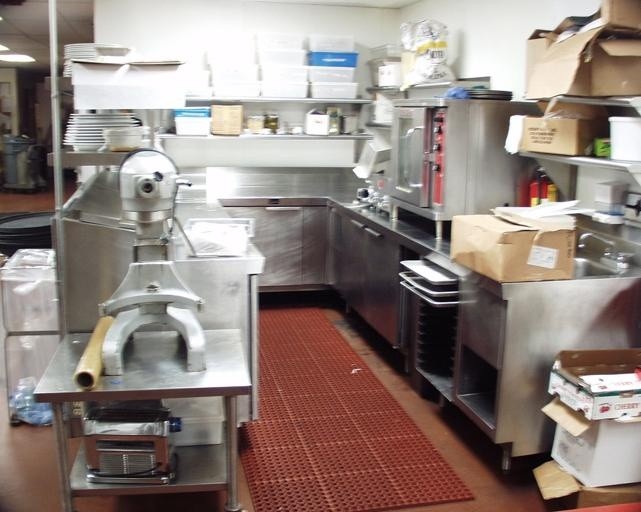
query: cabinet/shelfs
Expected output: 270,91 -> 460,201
388,99 -> 537,238
43,76 -> 156,167
326,208 -> 404,349
223,205 -> 326,287
398,256 -> 641,471
160,96 -> 373,139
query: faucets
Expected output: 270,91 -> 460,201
577,233 -> 616,257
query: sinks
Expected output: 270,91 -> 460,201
573,252 -> 624,279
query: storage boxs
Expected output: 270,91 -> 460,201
450,214 -> 576,283
3,334 -> 66,427
365,57 -> 402,88
532,348 -> 641,512
305,108 -> 331,136
522,1 -> 641,99
0,249 -> 59,333
504,115 -> 605,156
162,397 -> 226,447
173,105 -> 244,136
210,33 -> 359,97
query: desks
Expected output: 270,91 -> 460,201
33,328 -> 252,512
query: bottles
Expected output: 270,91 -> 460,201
548,184 -> 559,202
540,175 -> 548,199
265,112 -> 278,133
530,183 -> 539,207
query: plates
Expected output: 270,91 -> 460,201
64,113 -> 141,152
63,43 -> 103,78
465,88 -> 513,100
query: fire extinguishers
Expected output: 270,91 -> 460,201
530,167 -> 558,207
